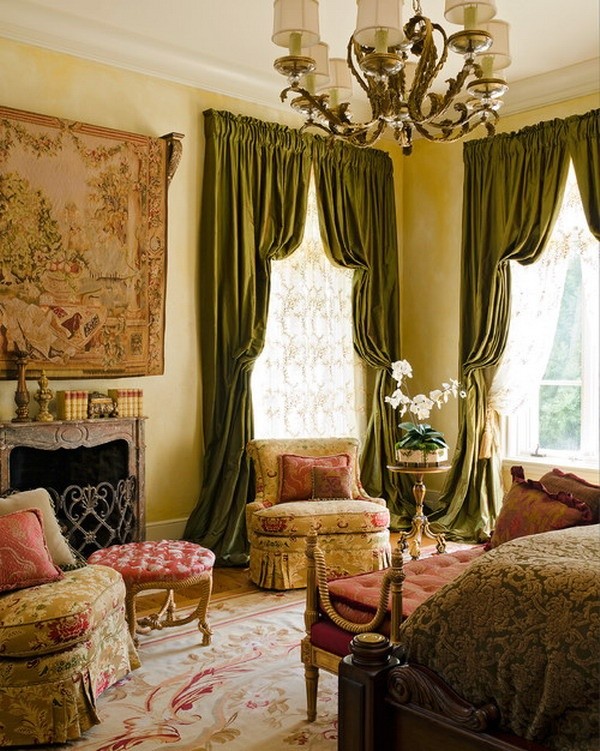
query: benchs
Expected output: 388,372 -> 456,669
299,526 -> 489,722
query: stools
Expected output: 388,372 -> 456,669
87,538 -> 216,647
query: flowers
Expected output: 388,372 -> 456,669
384,358 -> 467,451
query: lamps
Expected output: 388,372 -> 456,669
271,0 -> 512,155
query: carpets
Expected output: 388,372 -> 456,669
16,542 -> 478,751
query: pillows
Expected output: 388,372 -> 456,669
0,488 -> 87,594
482,465 -> 600,552
278,453 -> 353,502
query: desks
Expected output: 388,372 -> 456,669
386,465 -> 453,559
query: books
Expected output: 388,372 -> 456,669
56,390 -> 88,420
108,387 -> 144,418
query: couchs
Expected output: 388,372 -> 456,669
0,488 -> 143,746
244,438 -> 392,589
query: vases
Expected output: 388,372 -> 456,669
395,447 -> 449,464
